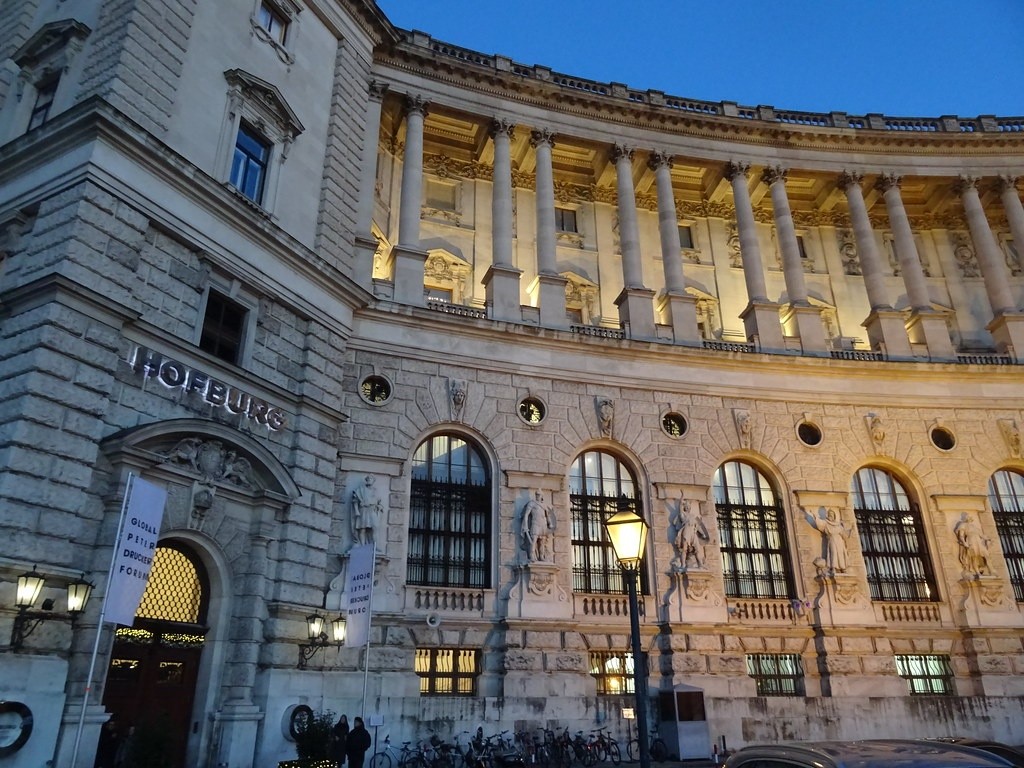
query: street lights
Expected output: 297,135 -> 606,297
603,492 -> 651,768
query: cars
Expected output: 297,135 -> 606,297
721,739 -> 1021,768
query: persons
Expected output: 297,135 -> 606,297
332,715 -> 371,768
353,474 -> 385,552
955,513 -> 997,576
522,489 -> 553,562
810,507 -> 854,573
673,487 -> 711,570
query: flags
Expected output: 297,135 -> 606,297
344,542 -> 376,647
104,475 -> 168,627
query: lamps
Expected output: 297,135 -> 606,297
10,564 -> 97,649
297,609 -> 347,670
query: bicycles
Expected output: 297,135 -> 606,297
368,726 -> 666,768
